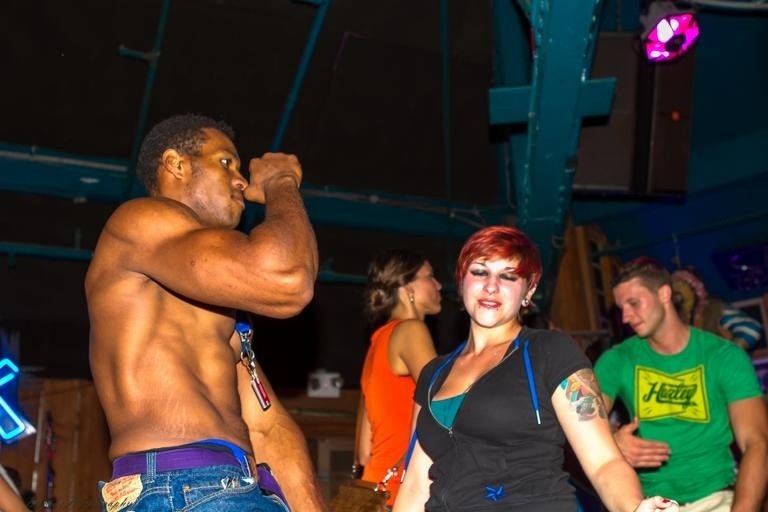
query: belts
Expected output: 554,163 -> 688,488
111,448 -> 292,511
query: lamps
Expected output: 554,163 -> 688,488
638,0 -> 700,64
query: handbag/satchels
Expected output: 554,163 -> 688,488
327,479 -> 388,512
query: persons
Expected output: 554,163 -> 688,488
537,511 -> 538,512
350,249 -> 444,507
673,264 -> 763,353
593,253 -> 767,512
0,474 -> 34,512
393,224 -> 683,512
83,113 -> 331,512
